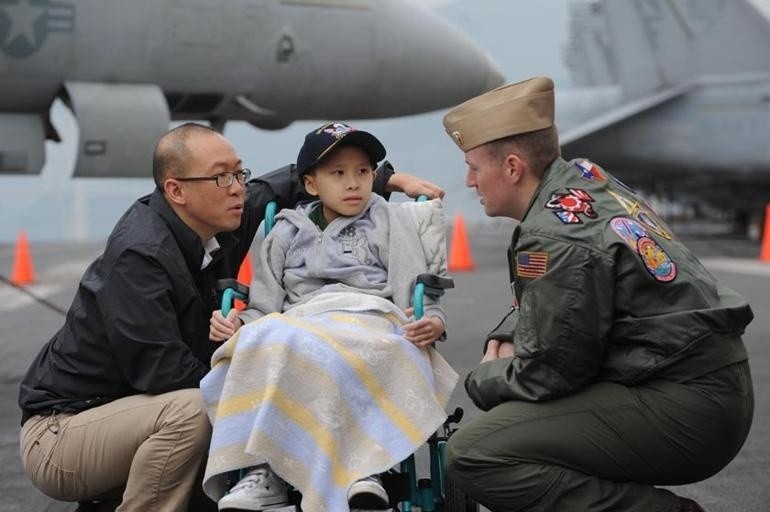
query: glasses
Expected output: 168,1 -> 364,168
173,168 -> 252,189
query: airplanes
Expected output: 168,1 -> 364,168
0,1 -> 508,179
553,0 -> 770,210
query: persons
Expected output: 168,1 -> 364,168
204,121 -> 452,512
16,121 -> 447,512
442,76 -> 755,511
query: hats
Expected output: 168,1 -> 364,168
294,117 -> 388,174
441,76 -> 555,152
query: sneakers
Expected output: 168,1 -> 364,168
217,467 -> 292,512
345,473 -> 390,511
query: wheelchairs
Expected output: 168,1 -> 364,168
218,194 -> 465,511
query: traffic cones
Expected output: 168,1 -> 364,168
446,211 -> 478,272
11,227 -> 34,284
758,203 -> 770,263
